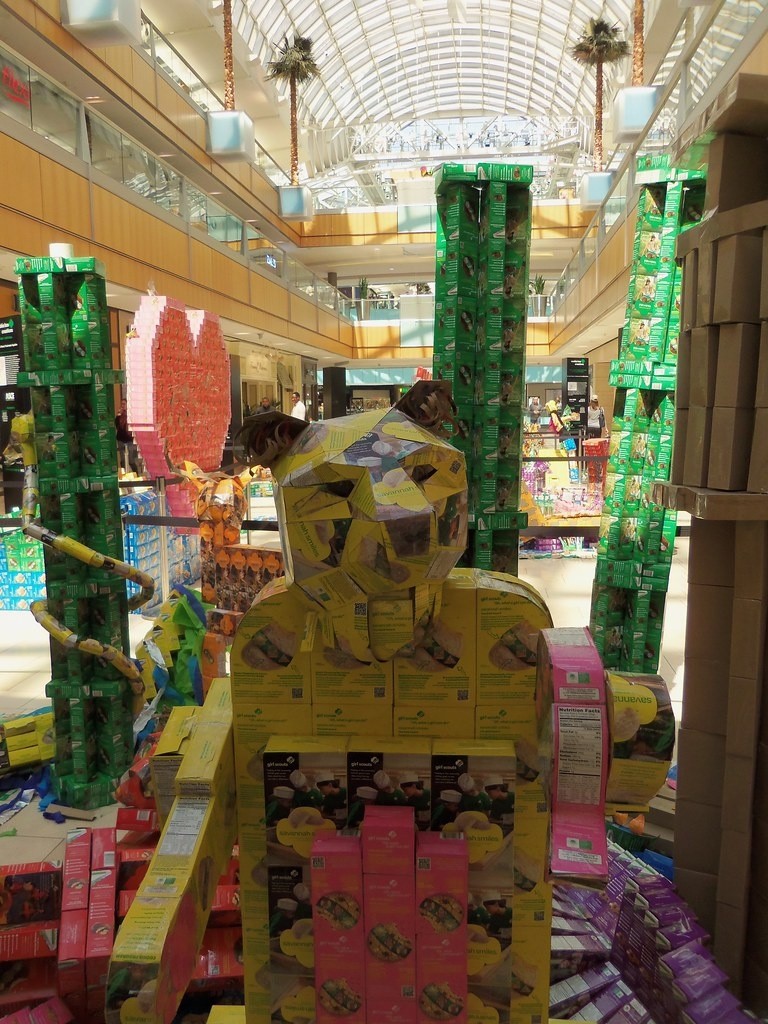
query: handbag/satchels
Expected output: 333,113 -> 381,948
599,407 -> 606,428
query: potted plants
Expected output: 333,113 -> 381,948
208,0 -> 258,161
614,0 -> 658,142
563,13 -> 634,211
263,26 -> 322,222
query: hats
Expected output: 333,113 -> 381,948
591,395 -> 599,402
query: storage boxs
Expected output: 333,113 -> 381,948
0,69 -> 768,1024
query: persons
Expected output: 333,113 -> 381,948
262,770 -> 519,827
587,394 -> 609,439
529,396 -> 545,424
255,396 -> 277,416
113,398 -> 139,479
290,392 -> 306,421
268,885 -> 517,938
